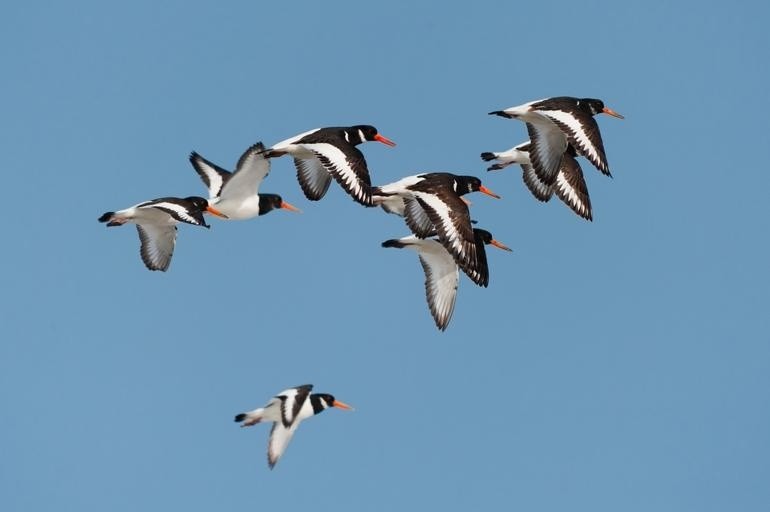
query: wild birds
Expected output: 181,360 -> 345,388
233,383 -> 352,471
97,196 -> 229,272
254,124 -> 396,209
487,95 -> 625,187
364,171 -> 500,270
480,134 -> 594,224
188,141 -> 305,222
380,225 -> 513,333
